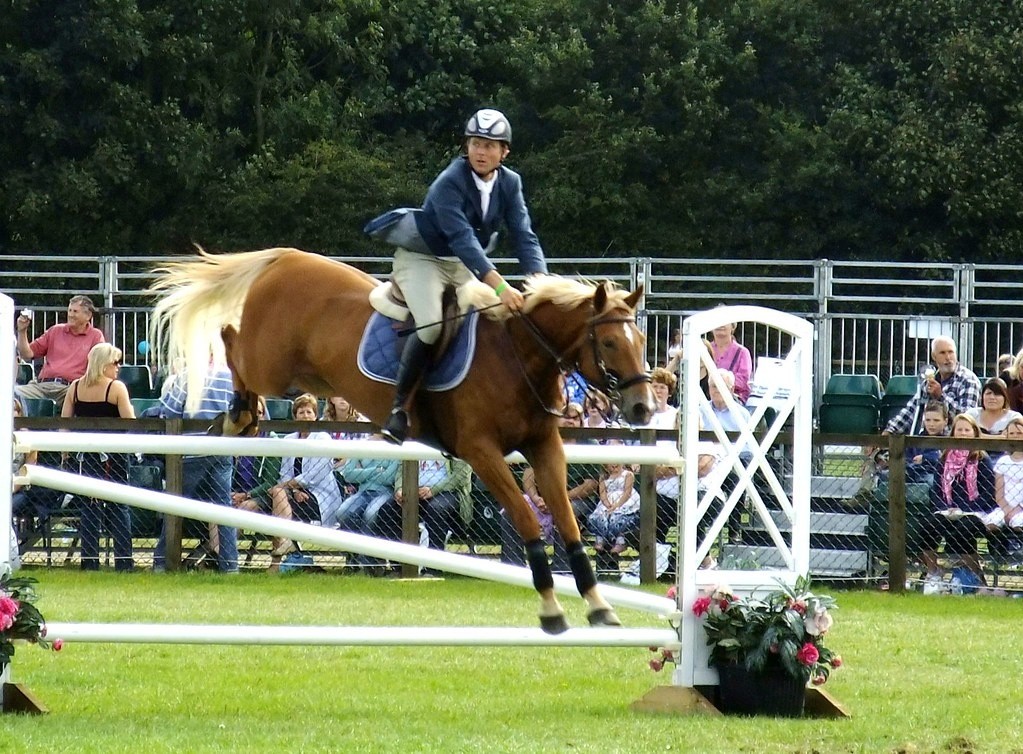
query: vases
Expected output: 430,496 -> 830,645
717,664 -> 812,717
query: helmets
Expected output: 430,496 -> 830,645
464,108 -> 512,144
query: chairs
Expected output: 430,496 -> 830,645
12,366 -> 1023,591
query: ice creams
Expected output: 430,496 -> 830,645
925,368 -> 935,382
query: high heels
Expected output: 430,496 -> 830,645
270,540 -> 298,557
265,556 -> 281,576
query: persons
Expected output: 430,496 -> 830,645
59,342 -> 138,574
9,295 -> 107,544
840,334 -> 1023,596
152,295 -> 474,576
499,304 -> 753,584
380,108 -> 551,446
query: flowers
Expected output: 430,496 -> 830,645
1,560 -> 61,671
650,571 -> 841,687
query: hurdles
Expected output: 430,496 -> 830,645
0,303 -> 851,721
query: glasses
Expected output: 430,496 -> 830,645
652,384 -> 668,390
109,359 -> 122,366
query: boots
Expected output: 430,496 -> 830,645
382,330 -> 438,447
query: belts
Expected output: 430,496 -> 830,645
36,377 -> 71,386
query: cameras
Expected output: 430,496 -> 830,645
20,308 -> 33,320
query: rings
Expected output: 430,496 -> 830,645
233,496 -> 235,499
928,386 -> 930,389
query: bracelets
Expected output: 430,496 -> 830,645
381,466 -> 383,472
495,281 -> 510,296
247,491 -> 251,499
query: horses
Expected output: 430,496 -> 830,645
149,243 -> 656,636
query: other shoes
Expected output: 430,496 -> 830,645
592,536 -> 719,585
903,567 -> 1022,602
